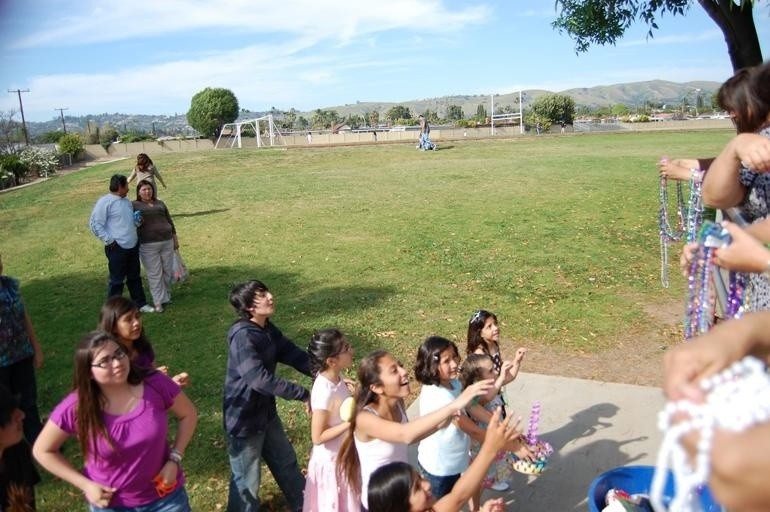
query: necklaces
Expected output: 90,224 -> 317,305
683,221 -> 756,338
650,161 -> 699,287
647,355 -> 766,512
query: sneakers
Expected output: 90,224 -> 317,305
140,304 -> 155,313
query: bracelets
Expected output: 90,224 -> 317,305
169,449 -> 185,465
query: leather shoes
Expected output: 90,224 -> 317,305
156,306 -> 165,312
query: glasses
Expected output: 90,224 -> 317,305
91,349 -> 126,368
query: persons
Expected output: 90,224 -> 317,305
89,174 -> 156,314
413,336 -> 542,499
416,115 -> 436,150
659,62 -> 770,186
368,407 -> 526,511
701,129 -> 770,218
677,218 -> 770,279
662,308 -> 769,512
32,330 -> 198,512
466,311 -> 506,419
303,328 -> 361,511
0,253 -> 63,452
99,296 -> 189,388
131,179 -> 179,312
128,153 -> 166,198
0,390 -> 41,512
223,280 -> 311,512
335,352 -> 495,512
458,348 -> 527,491
